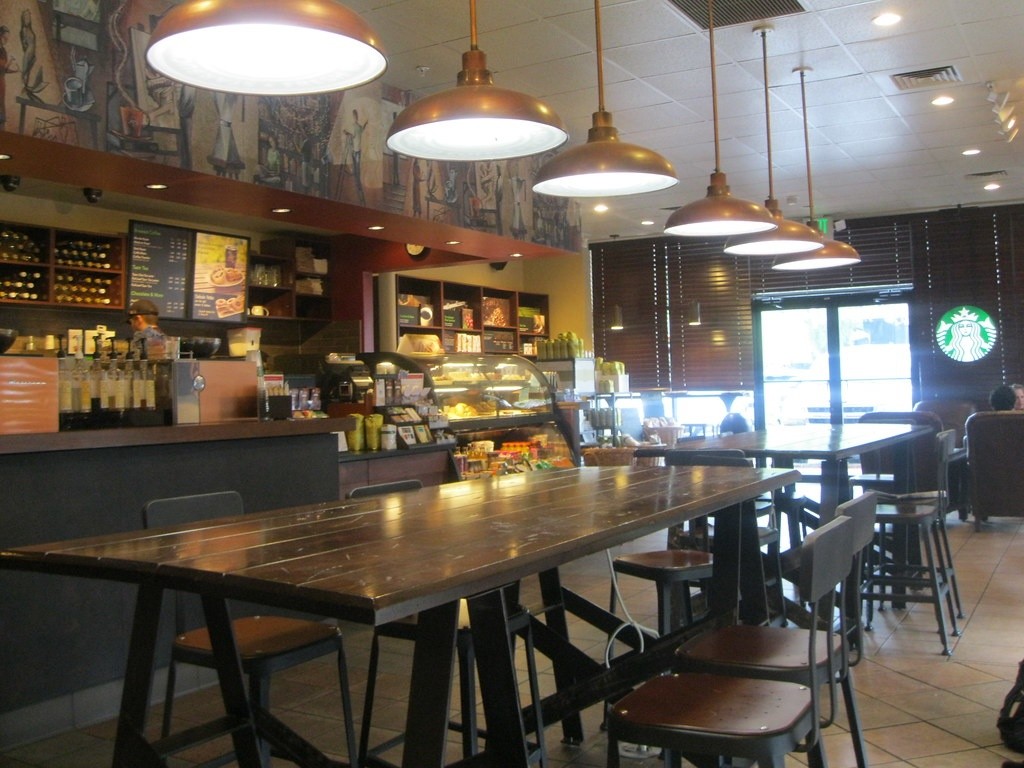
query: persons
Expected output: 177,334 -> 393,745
123,299 -> 168,359
990,382 -> 1024,412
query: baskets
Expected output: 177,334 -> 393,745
581,448 -> 637,466
641,426 -> 683,445
626,445 -> 667,466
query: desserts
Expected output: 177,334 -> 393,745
216,298 -> 243,315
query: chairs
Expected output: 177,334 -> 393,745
141,402 -> 1023,767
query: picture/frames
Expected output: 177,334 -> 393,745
127,219 -> 193,321
190,230 -> 251,324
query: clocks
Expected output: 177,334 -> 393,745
405,244 -> 427,257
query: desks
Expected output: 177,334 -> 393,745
633,423 -> 930,621
2,468 -> 803,768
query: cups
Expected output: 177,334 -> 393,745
246,350 -> 269,422
225,246 -> 237,268
421,308 -> 433,326
252,306 -> 270,317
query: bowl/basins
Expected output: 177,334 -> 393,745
181,337 -> 221,358
0,329 -> 19,354
209,270 -> 244,301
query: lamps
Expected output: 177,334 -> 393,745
385,1 -> 567,160
724,23 -> 826,260
769,65 -> 862,270
530,0 -> 678,196
663,0 -> 778,240
146,0 -> 389,96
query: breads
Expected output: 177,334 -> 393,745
211,266 -> 242,284
536,330 -> 582,359
595,357 -> 625,375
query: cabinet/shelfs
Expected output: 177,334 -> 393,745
2,220 -> 582,499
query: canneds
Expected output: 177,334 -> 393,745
225,245 -> 237,268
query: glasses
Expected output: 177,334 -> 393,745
126,314 -> 143,325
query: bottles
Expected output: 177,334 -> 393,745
1,228 -> 115,304
149,337 -> 179,360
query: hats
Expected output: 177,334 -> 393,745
119,306 -> 159,323
